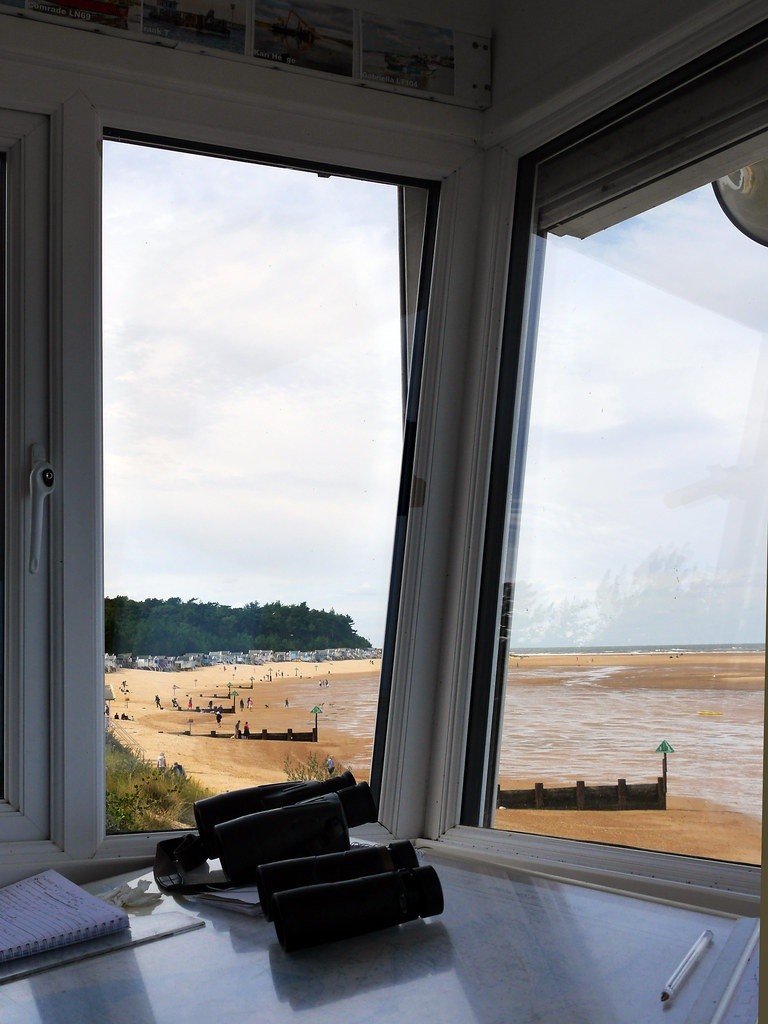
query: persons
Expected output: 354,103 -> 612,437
327,755 -> 335,776
173,762 -> 187,779
105,706 -> 136,720
155,660 -> 374,739
157,752 -> 166,771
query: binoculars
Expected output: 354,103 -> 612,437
256,838 -> 445,954
154,770 -> 378,895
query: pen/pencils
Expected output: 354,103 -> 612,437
660,929 -> 713,1002
